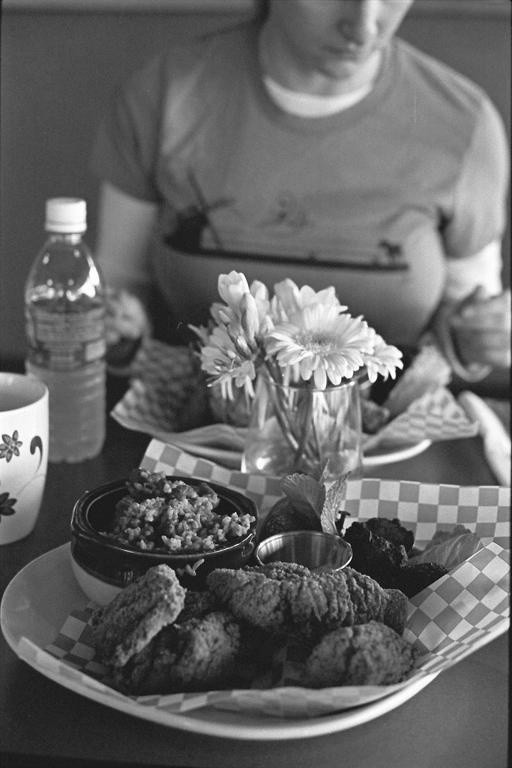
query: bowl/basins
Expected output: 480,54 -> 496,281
68,476 -> 256,610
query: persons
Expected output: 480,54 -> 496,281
79,0 -> 510,404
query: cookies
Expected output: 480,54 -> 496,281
86,561 -> 419,693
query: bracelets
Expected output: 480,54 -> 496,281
441,314 -> 493,382
104,329 -> 151,377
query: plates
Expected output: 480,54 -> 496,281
1,539 -> 443,742
152,434 -> 431,468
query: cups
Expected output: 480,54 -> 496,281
256,530 -> 354,576
0,372 -> 50,544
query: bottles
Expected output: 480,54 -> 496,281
22,197 -> 106,465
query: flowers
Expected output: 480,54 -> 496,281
187,270 -> 404,475
